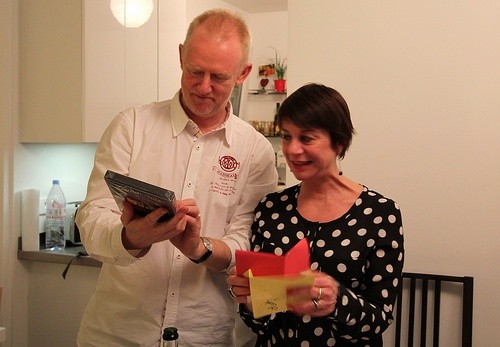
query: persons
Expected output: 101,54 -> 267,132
221,84 -> 405,347
76,9 -> 279,347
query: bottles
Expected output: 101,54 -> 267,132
163,327 -> 178,347
274,103 -> 281,136
45,180 -> 66,251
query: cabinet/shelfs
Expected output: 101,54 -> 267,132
248,91 -> 287,188
20,0 -> 158,145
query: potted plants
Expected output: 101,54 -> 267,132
268,47 -> 287,93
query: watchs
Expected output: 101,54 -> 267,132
189,237 -> 213,265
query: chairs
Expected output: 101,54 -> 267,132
395,272 -> 473,347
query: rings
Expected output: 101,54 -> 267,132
311,300 -> 320,310
315,287 -> 322,301
196,214 -> 201,220
227,286 -> 238,298
